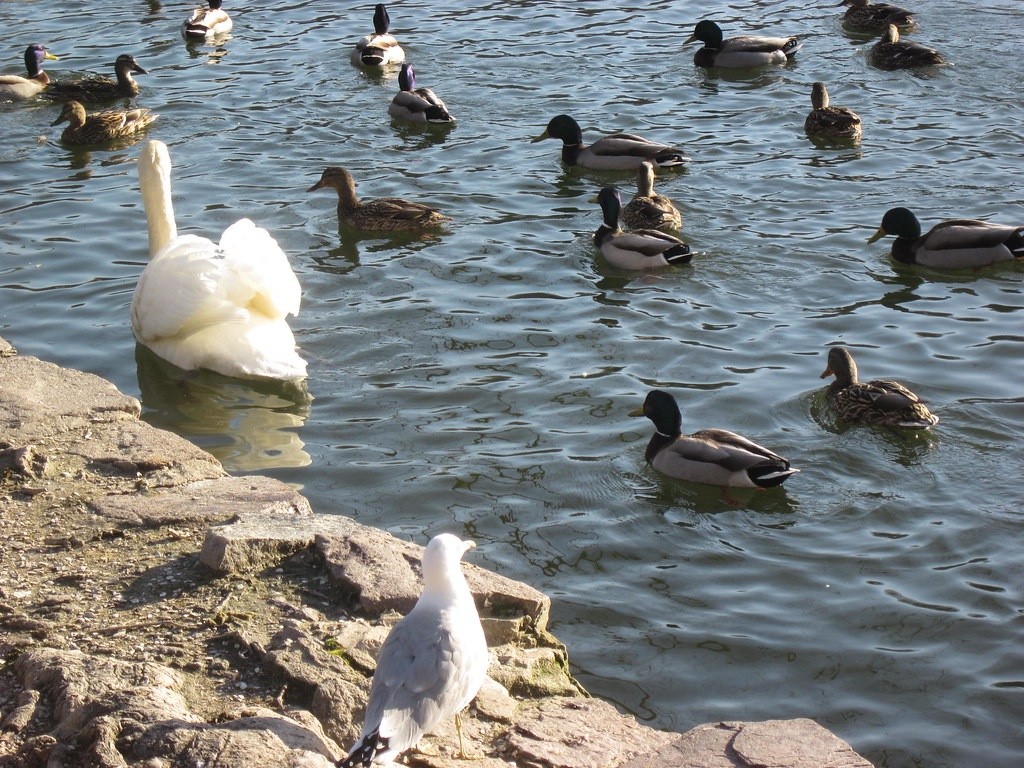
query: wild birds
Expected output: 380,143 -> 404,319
682,20 -> 802,68
308,164 -> 452,231
46,54 -> 148,105
837,0 -> 920,33
867,24 -> 950,70
628,390 -> 800,488
619,160 -> 683,233
868,207 -> 1024,268
530,112 -> 692,173
0,44 -> 58,101
391,62 -> 457,125
336,532 -> 489,768
181,0 -> 232,40
587,188 -> 691,269
50,98 -> 158,144
818,346 -> 941,430
805,82 -> 862,145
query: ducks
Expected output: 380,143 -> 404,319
351,3 -> 404,67
130,142 -> 309,386
